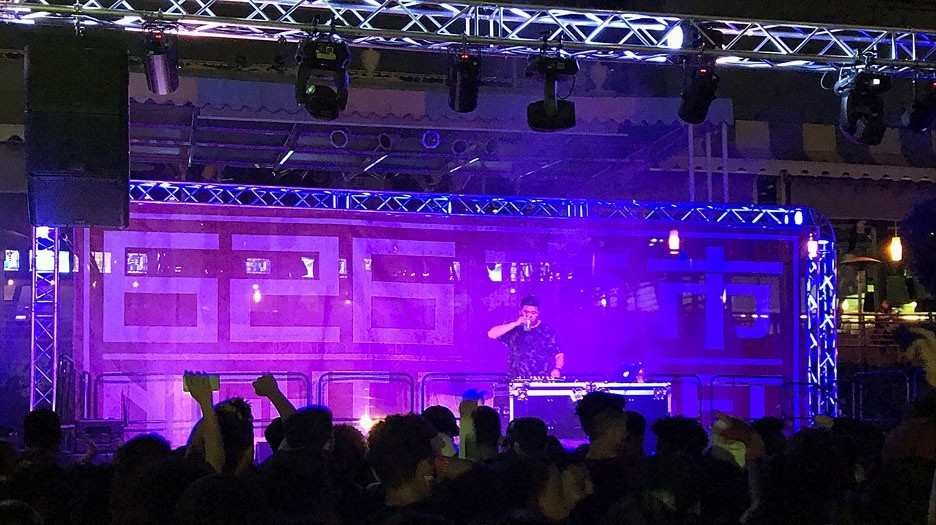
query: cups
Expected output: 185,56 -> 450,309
524,319 -> 531,331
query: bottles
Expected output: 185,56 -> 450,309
636,362 -> 645,382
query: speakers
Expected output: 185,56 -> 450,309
73,419 -> 123,453
19,24 -> 133,228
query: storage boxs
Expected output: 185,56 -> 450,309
508,383 -> 671,459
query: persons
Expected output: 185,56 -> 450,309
486,295 -> 565,383
0,369 -> 936,525
875,298 -> 898,326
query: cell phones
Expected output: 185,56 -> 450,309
183,372 -> 220,392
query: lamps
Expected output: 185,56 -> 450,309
295,32 -> 353,121
446,42 -> 481,114
677,56 -> 721,124
141,20 -> 180,95
524,56 -> 581,132
668,227 -> 681,255
891,221 -> 902,262
834,64 -> 893,147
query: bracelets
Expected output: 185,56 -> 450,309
441,457 -> 451,474
555,367 -> 561,372
